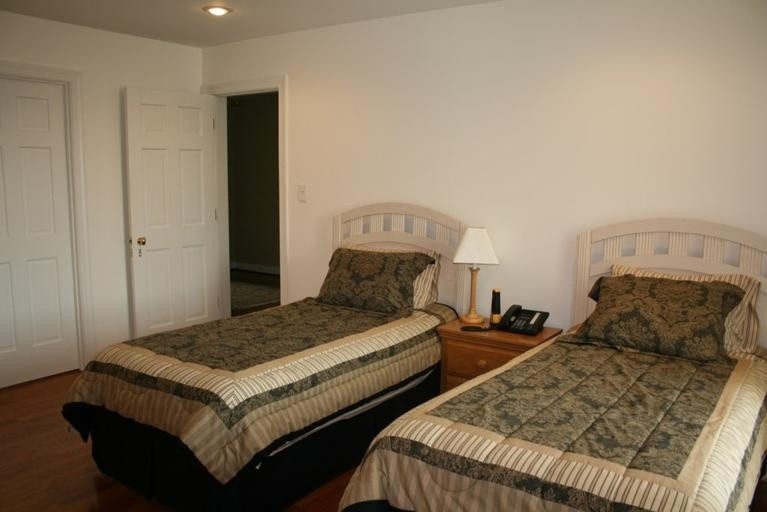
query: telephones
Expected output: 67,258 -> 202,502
497,305 -> 549,336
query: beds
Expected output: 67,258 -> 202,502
59,201 -> 467,512
338,216 -> 767,512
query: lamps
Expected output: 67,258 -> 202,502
452,227 -> 500,324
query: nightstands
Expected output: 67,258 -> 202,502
437,316 -> 563,392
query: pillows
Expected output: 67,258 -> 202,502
609,261 -> 761,360
575,274 -> 744,365
339,239 -> 442,312
316,247 -> 436,317
